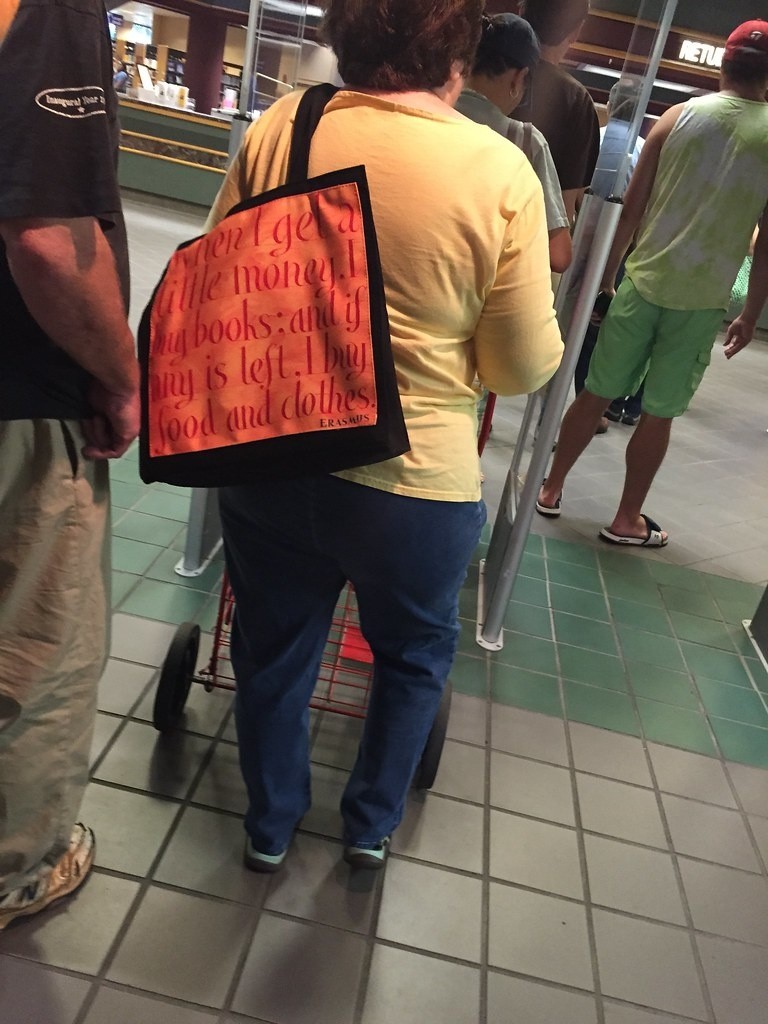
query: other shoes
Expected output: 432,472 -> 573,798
622,413 -> 640,425
596,417 -> 608,434
606,403 -> 623,423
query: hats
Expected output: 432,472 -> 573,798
479,12 -> 537,109
722,17 -> 767,63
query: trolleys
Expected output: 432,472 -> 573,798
148,538 -> 457,791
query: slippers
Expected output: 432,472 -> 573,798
600,514 -> 669,547
537,478 -> 562,516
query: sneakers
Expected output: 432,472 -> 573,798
245,835 -> 292,872
0,823 -> 99,927
344,831 -> 393,873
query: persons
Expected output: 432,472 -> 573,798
453,12 -> 574,272
574,79 -> 645,435
505,0 -> 601,226
1,1 -> 143,931
203,0 -> 565,882
603,379 -> 647,428
113,61 -> 128,93
535,17 -> 768,548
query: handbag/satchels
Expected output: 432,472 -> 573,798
139,83 -> 411,486
